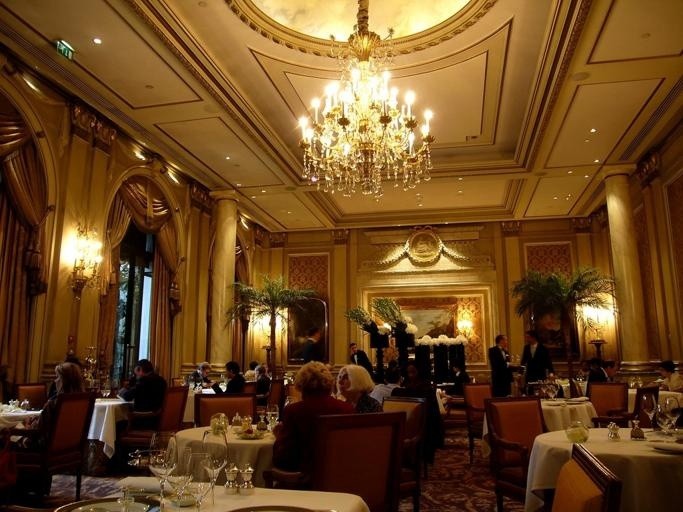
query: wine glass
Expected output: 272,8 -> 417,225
641,393 -> 656,432
179,374 -> 187,387
105,386 -> 110,397
539,379 -> 560,400
183,452 -> 214,512
148,431 -> 178,512
663,396 -> 681,433
256,406 -> 267,430
100,385 -> 106,398
656,404 -> 672,434
196,379 -> 201,390
166,447 -> 193,512
200,430 -> 228,512
285,396 -> 297,406
266,404 -> 279,432
188,375 -> 195,391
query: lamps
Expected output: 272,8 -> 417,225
292,0 -> 435,200
61,220 -> 104,303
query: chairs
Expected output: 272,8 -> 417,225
586,381 -> 628,425
1,393 -> 102,501
171,378 -> 186,389
591,386 -> 659,428
383,397 -> 430,482
194,393 -> 258,428
463,384 -> 493,464
549,442 -> 621,512
255,380 -> 284,414
307,410 -> 422,512
240,381 -> 258,395
482,395 -> 553,512
285,383 -> 304,401
17,386 -> 46,409
118,385 -> 189,470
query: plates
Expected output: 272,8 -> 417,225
54,496 -> 164,512
228,506 -> 338,512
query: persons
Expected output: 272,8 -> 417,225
242,361 -> 258,382
588,359 -> 618,384
272,360 -> 355,472
445,360 -> 470,397
349,342 -> 373,380
369,368 -> 407,405
118,358 -> 167,431
591,358 -> 602,369
210,361 -> 245,395
187,362 -> 213,386
657,361 -> 683,393
578,360 -> 593,381
0,362 -> 90,505
256,367 -> 272,395
337,364 -> 383,413
488,334 -> 526,398
521,331 -> 555,395
303,328 -> 325,365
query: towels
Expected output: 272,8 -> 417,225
645,438 -> 680,454
115,477 -> 179,496
